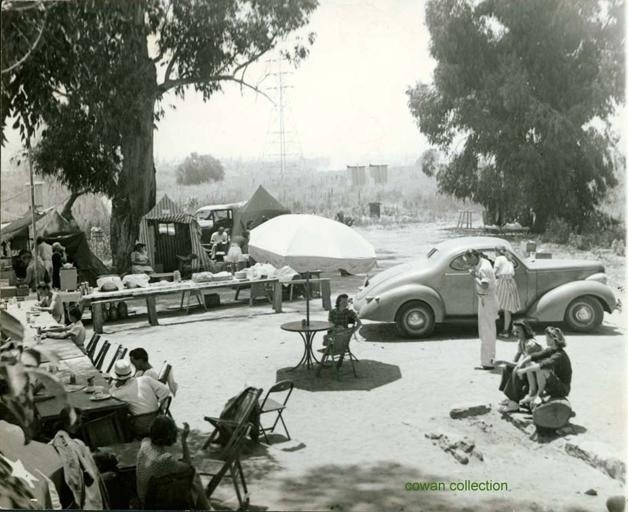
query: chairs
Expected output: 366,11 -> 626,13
200,386 -> 264,458
326,316 -> 359,365
315,332 -> 357,380
0,299 -> 203,511
254,379 -> 294,445
199,423 -> 255,511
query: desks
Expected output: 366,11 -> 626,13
280,320 -> 335,374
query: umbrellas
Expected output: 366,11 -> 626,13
248,214 -> 376,326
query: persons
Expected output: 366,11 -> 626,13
35,282 -> 65,323
493,244 -> 521,338
131,239 -> 154,274
136,415 -> 216,512
109,359 -> 170,434
52,242 -> 67,288
465,248 -> 500,370
498,319 -> 545,403
42,305 -> 86,349
129,348 -> 159,380
512,326 -> 572,406
328,294 -> 362,333
210,227 -> 229,260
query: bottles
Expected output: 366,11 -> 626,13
174,271 -> 181,285
301,318 -> 306,326
80,281 -> 89,295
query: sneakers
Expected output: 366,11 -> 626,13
500,393 -> 542,410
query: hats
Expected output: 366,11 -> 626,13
111,359 -> 135,380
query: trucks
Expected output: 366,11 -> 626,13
160,205 -> 236,249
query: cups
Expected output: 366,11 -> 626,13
93,391 -> 103,397
46,364 -> 56,375
69,374 -> 75,384
87,382 -> 95,391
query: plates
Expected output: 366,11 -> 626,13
89,394 -> 111,401
234,272 -> 246,279
83,386 -> 104,392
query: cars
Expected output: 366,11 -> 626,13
351,241 -> 616,335
